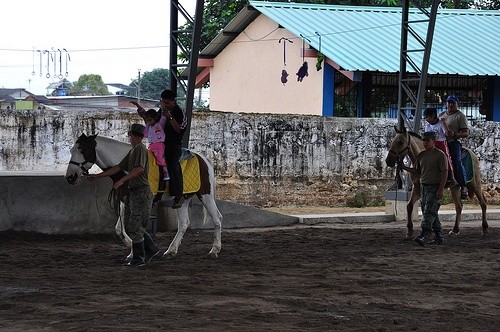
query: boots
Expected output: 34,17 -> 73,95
123,240 -> 145,267
143,232 -> 159,263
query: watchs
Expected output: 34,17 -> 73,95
454,132 -> 457,137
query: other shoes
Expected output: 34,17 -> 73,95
172,195 -> 184,209
415,237 -> 424,246
162,171 -> 170,180
428,238 -> 443,244
460,186 -> 468,198
449,179 -> 457,186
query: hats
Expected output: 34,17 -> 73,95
421,131 -> 437,139
446,95 -> 459,104
128,124 -> 145,139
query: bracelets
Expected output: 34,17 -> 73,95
168,116 -> 173,121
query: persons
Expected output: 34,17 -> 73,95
423,95 -> 469,199
128,89 -> 188,209
87,123 -> 160,269
401,131 -> 449,247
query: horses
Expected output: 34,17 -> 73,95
385,114 -> 489,240
65,132 -> 224,266
